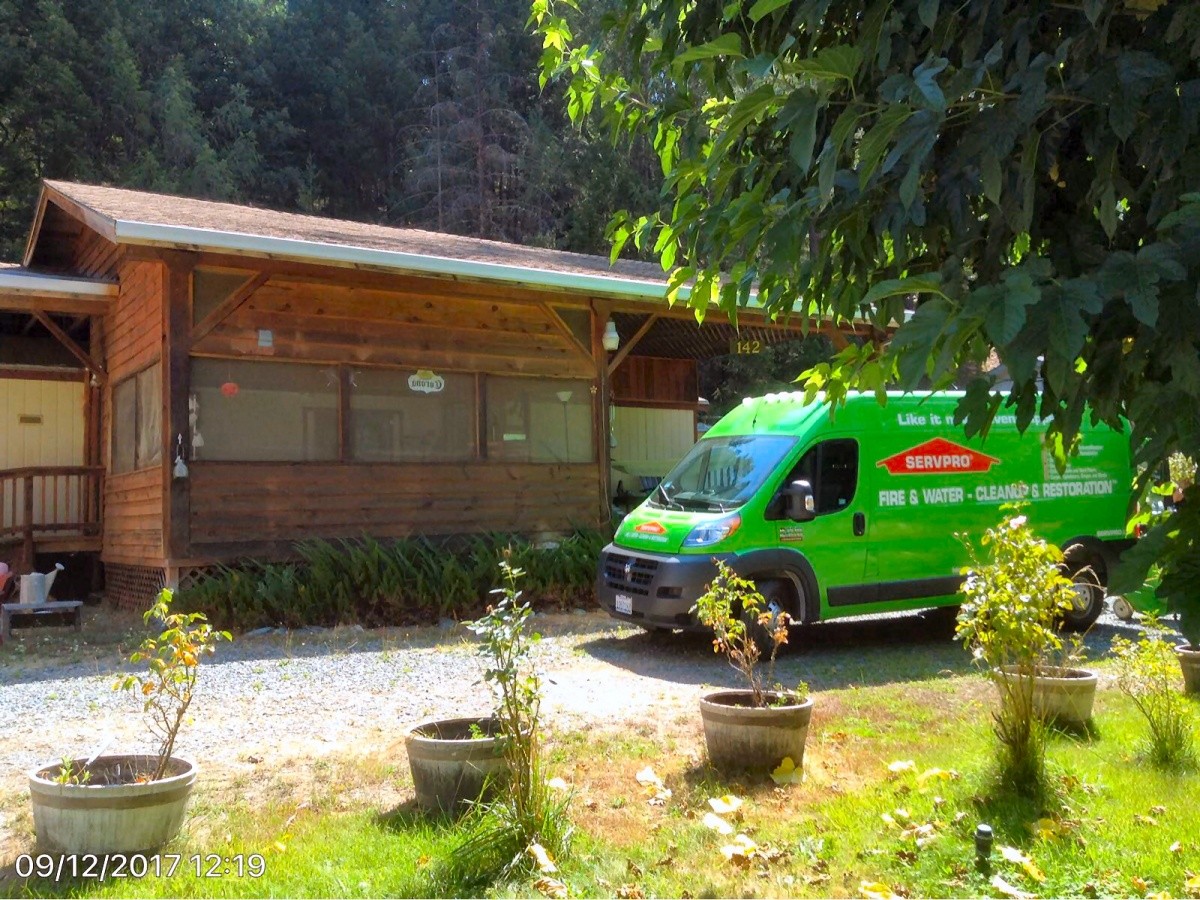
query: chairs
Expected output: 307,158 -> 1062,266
815,445 -> 855,512
640,475 -> 661,498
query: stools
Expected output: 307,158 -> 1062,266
0,600 -> 83,642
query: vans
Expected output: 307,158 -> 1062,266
594,389 -> 1146,663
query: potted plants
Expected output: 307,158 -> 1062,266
28,588 -> 235,869
687,551 -> 814,783
404,716 -> 533,817
993,613 -> 1100,731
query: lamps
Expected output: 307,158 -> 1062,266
555,391 -> 572,462
174,459 -> 188,482
601,319 -> 619,351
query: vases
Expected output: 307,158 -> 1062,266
1174,645 -> 1200,693
